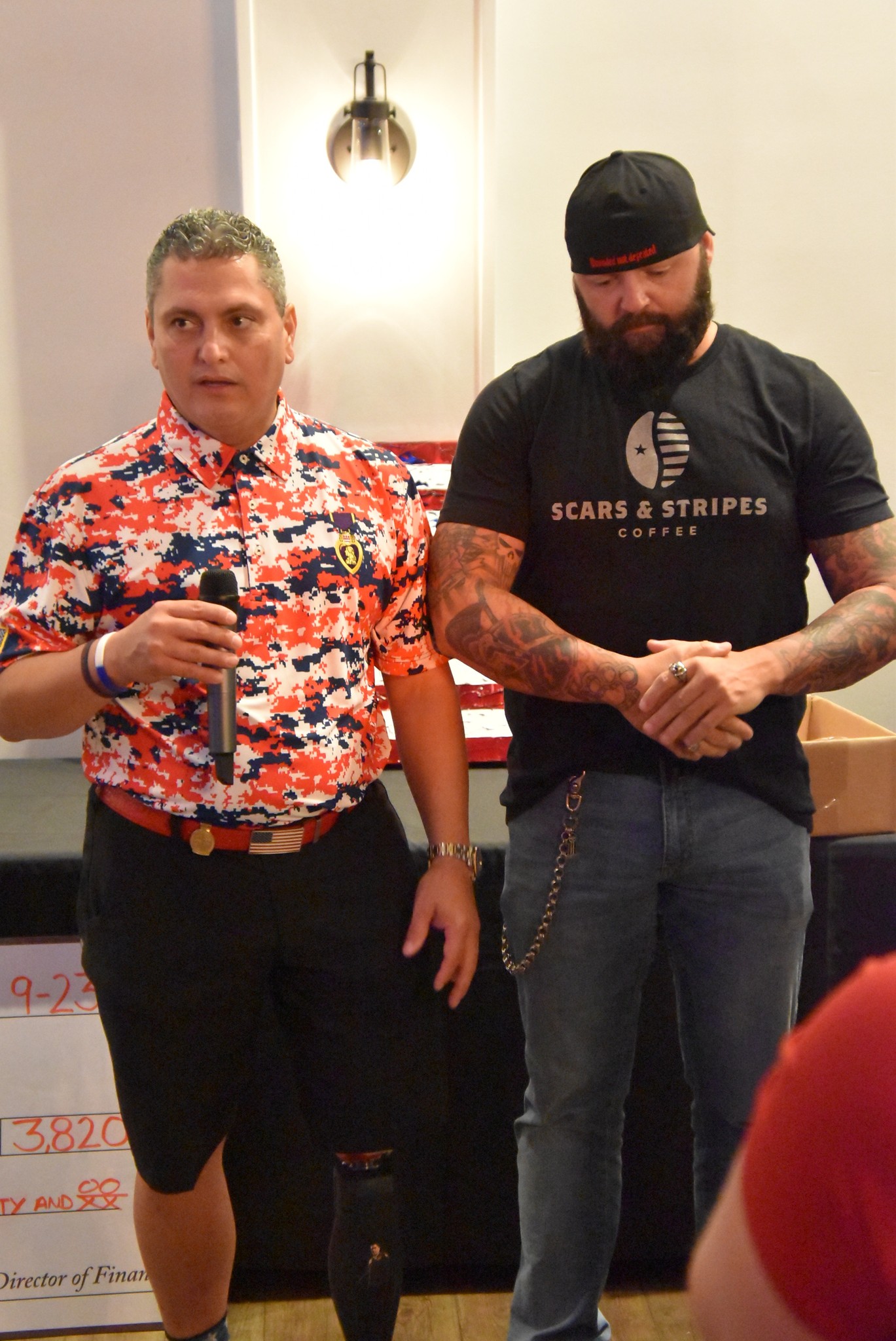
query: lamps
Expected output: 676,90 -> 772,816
331,48 -> 414,199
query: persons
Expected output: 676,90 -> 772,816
431,150 -> 896,1341
0,203 -> 482,1341
685,951 -> 896,1341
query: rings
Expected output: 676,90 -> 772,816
669,662 -> 686,683
685,743 -> 701,756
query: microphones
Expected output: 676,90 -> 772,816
197,568 -> 241,786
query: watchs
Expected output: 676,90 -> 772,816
427,843 -> 482,883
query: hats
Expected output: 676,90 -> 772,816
564,150 -> 716,275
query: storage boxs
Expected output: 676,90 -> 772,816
797,694 -> 896,837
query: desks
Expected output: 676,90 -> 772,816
1,758 -> 896,1310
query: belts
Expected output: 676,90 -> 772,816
95,783 -> 357,855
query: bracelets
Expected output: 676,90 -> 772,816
80,640 -> 118,698
95,631 -> 132,693
